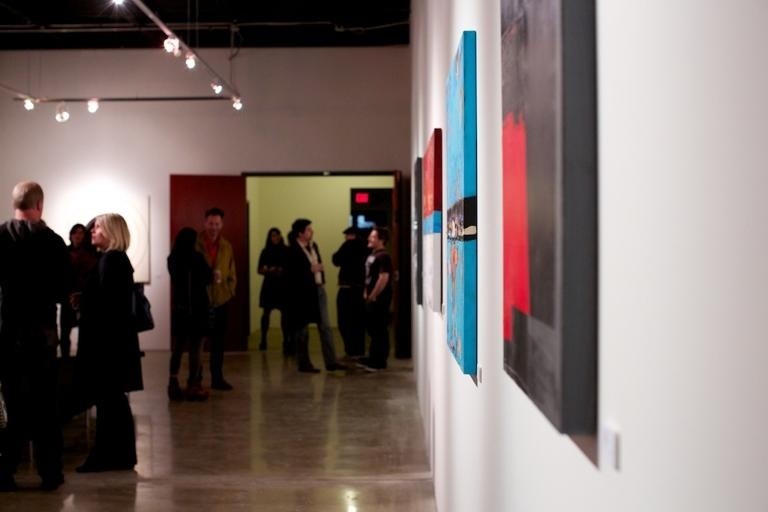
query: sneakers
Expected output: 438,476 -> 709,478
211,380 -> 233,391
325,362 -> 344,371
359,356 -> 386,368
298,362 -> 321,373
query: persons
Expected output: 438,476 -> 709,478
60,222 -> 91,357
69,210 -> 144,474
0,177 -> 72,491
194,206 -> 238,391
353,225 -> 395,373
330,227 -> 366,361
257,226 -> 292,350
287,217 -> 352,374
165,227 -> 217,401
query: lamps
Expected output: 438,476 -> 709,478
24,99 -> 33,111
131,0 -> 244,113
87,97 -> 99,113
54,102 -> 70,123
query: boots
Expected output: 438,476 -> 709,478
167,377 -> 183,401
185,376 -> 209,402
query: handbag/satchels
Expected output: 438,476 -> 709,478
133,282 -> 154,332
